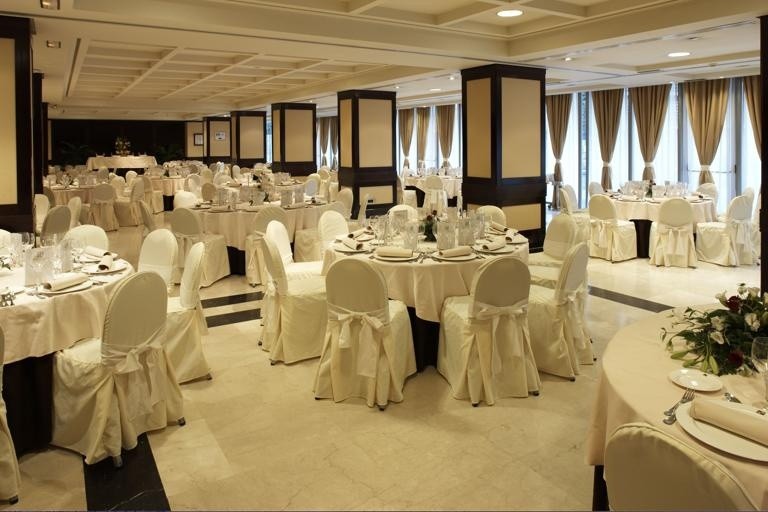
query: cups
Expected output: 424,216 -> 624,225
23,233 -> 36,253
432,223 -> 437,240
369,215 -> 380,233
386,216 -> 400,242
750,335 -> 768,410
46,232 -> 72,272
403,221 -> 417,250
294,183 -> 306,202
378,214 -> 387,246
248,188 -> 258,207
280,186 -> 293,206
668,368 -> 725,392
438,221 -> 456,250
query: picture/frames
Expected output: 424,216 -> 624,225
194,133 -> 204,145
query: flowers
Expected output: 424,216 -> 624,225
661,283 -> 768,376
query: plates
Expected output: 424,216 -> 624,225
189,204 -> 212,209
675,400 -> 768,463
471,245 -> 515,254
78,251 -> 119,263
431,250 -> 477,261
80,261 -> 128,274
207,209 -> 235,213
373,253 -> 419,261
334,242 -> 376,253
305,201 -> 327,205
335,235 -> 376,241
492,236 -> 529,245
37,279 -> 94,294
0,285 -> 27,296
486,227 -> 520,235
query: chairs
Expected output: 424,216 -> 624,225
696,183 -> 718,221
319,170 -> 329,204
189,164 -> 199,175
139,199 -> 172,250
447,167 -> 457,175
127,154 -> 134,157
178,168 -> 189,177
111,155 -> 121,157
405,169 -> 415,177
423,175 -> 447,209
213,171 -> 222,185
209,163 -> 217,172
184,174 -> 202,200
258,220 -> 323,346
0,326 -> 21,505
239,183 -> 258,204
173,191 -> 198,210
319,211 -> 349,256
163,162 -> 169,170
308,174 -> 321,195
231,164 -> 241,180
438,256 -> 542,407
125,170 -> 137,185
564,185 -> 589,213
560,188 -> 591,244
427,167 -> 437,175
75,165 -> 86,176
397,175 -> 417,208
69,169 -> 79,179
87,175 -> 96,185
476,205 -> 507,227
123,178 -> 137,197
329,174 -> 338,203
245,206 -> 290,287
97,155 -> 104,158
605,423 -> 758,512
113,177 -> 145,226
457,191 -> 462,208
80,183 -> 119,231
145,176 -> 164,214
68,196 -> 82,229
166,243 -> 212,385
138,229 -> 179,294
293,202 -> 348,262
529,214 -> 577,266
218,175 -> 231,185
529,242 -> 596,381
112,176 -> 125,197
199,164 -> 207,174
255,163 -> 266,172
589,194 -> 637,262
139,155 -> 147,156
241,167 -> 250,174
312,258 -> 416,412
0,228 -> 11,254
649,198 -> 697,268
717,188 -> 754,223
60,225 -> 109,251
169,167 -> 177,177
262,236 -> 326,365
47,174 -> 56,185
167,207 -> 231,288
40,205 -> 72,246
109,172 -> 116,183
589,182 -> 605,197
388,205 -> 418,219
96,166 -> 109,186
152,164 -> 162,177
337,188 -> 353,221
200,168 -> 213,183
697,196 -> 760,266
65,165 -> 74,172
202,183 -> 216,202
51,271 -> 185,468
303,179 -> 318,196
33,194 -> 50,246
348,193 -> 369,232
79,175 -> 86,184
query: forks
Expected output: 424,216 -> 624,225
662,387 -> 695,417
